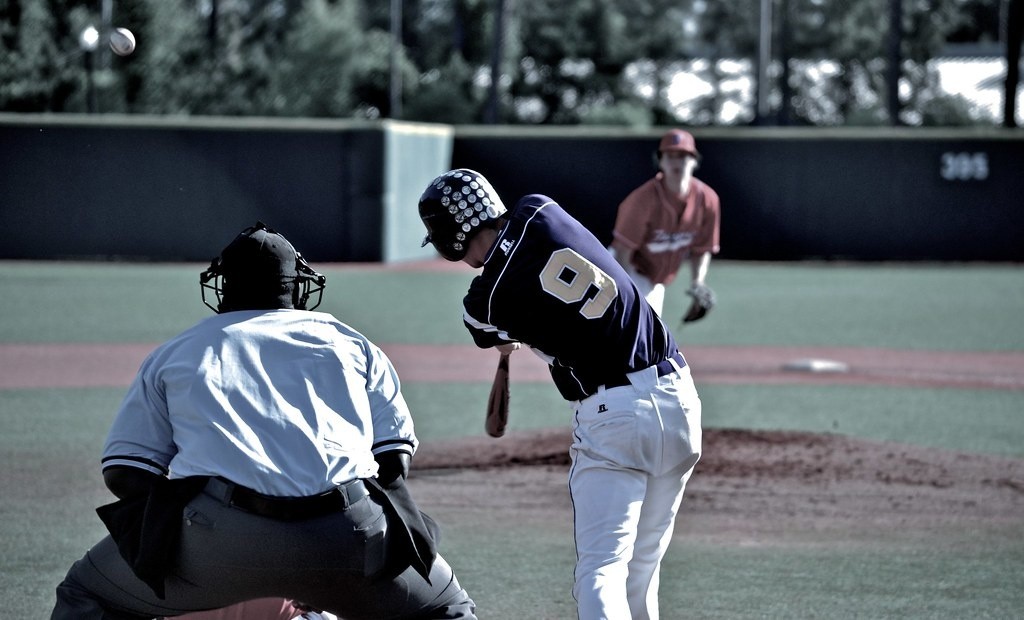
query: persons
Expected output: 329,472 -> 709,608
608,130 -> 721,324
51,221 -> 477,620
418,168 -> 702,620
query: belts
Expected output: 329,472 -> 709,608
587,355 -> 686,395
204,477 -> 371,519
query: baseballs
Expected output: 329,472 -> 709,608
108,25 -> 136,56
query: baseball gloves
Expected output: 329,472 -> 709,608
682,283 -> 719,325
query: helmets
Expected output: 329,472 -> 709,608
418,168 -> 509,261
660,130 -> 697,157
199,222 -> 325,316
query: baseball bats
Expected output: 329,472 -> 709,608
484,355 -> 512,438
